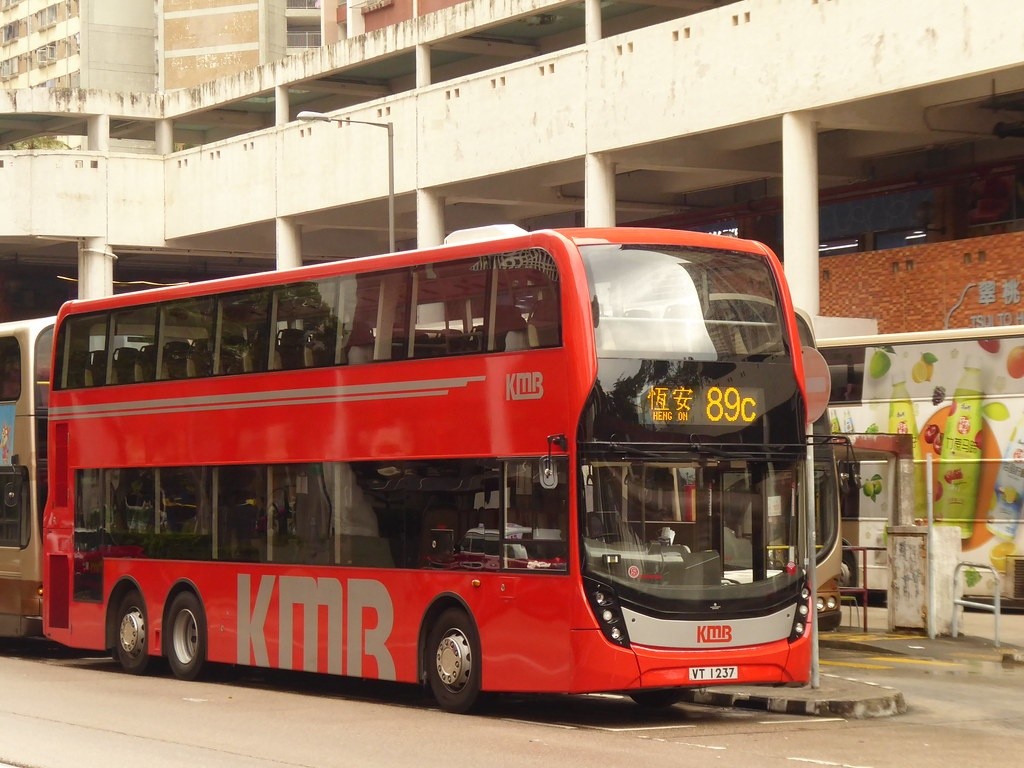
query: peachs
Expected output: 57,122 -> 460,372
1007,346 -> 1024,379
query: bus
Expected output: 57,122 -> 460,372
341,291 -> 842,634
0,313 -> 224,643
39,222 -> 862,717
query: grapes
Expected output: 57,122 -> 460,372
964,566 -> 983,588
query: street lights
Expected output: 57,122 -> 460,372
298,111 -> 396,252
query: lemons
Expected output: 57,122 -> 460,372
989,542 -> 1018,572
912,352 -> 934,384
863,481 -> 882,497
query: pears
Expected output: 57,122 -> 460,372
870,346 -> 892,379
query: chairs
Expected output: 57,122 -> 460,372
111,347 -> 138,384
83,350 -> 107,386
346,342 -> 374,364
465,324 -> 484,350
186,339 -> 211,377
134,345 -> 157,382
436,329 -> 465,353
243,331 -> 268,371
274,329 -> 303,370
527,298 -> 559,347
303,329 -> 321,366
505,330 -> 528,352
211,336 -> 232,374
160,341 -> 191,379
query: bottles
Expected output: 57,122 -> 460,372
829,409 -> 840,434
984,413 -> 1024,538
887,371 -> 930,521
842,408 -> 856,447
931,353 -> 985,539
